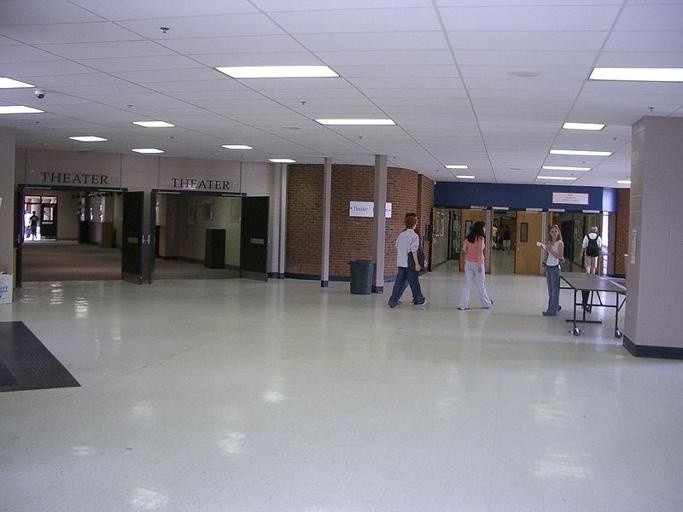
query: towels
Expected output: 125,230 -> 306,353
347,260 -> 376,295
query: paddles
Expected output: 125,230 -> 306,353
0,320 -> 82,392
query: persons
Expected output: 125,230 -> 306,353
502,224 -> 512,255
540,225 -> 564,316
396,213 -> 425,305
43,211 -> 48,220
388,216 -> 426,308
24,211 -> 33,238
29,211 -> 39,241
457,221 -> 493,310
580,226 -> 602,275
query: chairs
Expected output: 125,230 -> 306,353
459,305 -> 471,310
543,305 -> 562,317
390,300 -> 403,309
482,298 -> 493,309
412,296 -> 428,306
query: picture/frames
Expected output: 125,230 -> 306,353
557,271 -> 625,337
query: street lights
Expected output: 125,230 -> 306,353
409,249 -> 425,271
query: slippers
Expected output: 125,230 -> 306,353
586,234 -> 600,258
